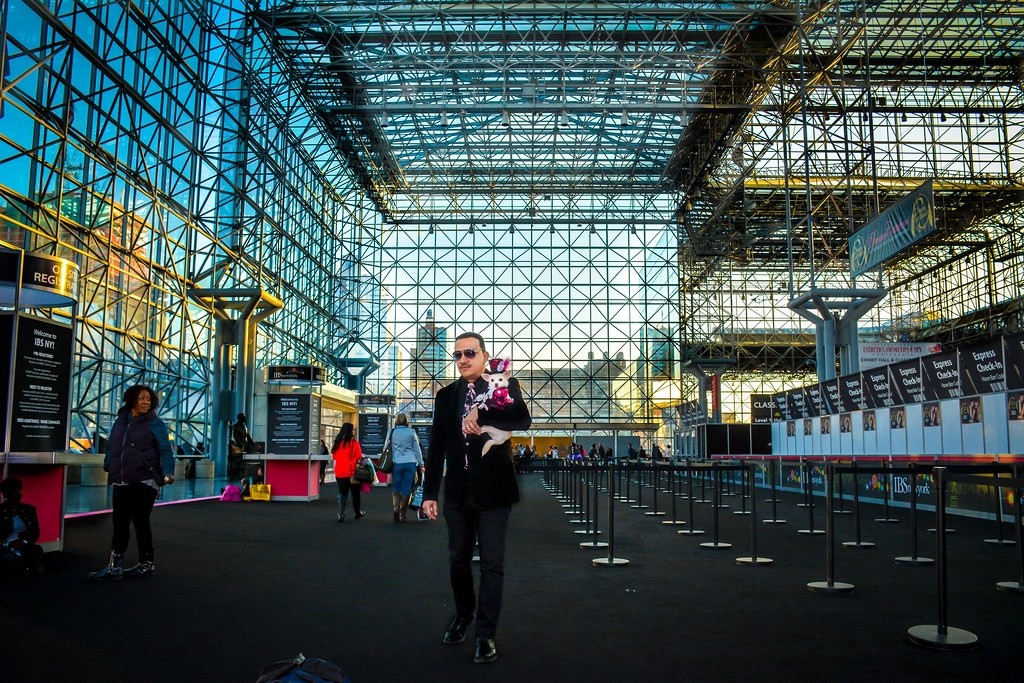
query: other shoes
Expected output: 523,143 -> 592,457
355,511 -> 365,519
338,516 -> 344,522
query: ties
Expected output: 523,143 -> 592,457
461,383 -> 477,470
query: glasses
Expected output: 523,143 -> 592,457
452,349 -> 484,361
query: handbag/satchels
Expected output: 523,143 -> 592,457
409,473 -> 424,510
249,481 -> 271,503
379,429 -> 395,473
354,457 -> 379,493
152,468 -> 164,487
220,485 -> 241,502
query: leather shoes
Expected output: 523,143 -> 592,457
443,613 -> 476,643
473,638 -> 497,663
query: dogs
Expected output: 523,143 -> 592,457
478,369 -> 513,457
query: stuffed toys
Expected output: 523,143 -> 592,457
477,357 -> 512,411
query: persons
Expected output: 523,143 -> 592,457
419,331 -> 532,659
320,439 -> 329,485
788,418 -> 830,435
231,412 -> 261,455
568,441 -> 613,461
253,465 -> 264,484
0,476 -> 44,577
866,412 -> 874,431
665,444 -> 673,457
89,385 -> 175,577
967,400 -> 980,424
627,442 -> 637,460
509,441 -> 536,475
382,412 -> 426,525
639,445 -> 646,460
1015,393 -> 1024,420
844,414 -> 850,432
652,443 -> 662,462
929,404 -> 938,426
330,421 -> 367,521
896,408 -> 904,429
546,446 -> 559,460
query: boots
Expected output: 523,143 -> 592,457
392,492 -> 401,521
123,547 -> 155,577
399,495 -> 409,523
88,550 -> 123,580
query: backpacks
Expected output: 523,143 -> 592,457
255,652 -> 354,683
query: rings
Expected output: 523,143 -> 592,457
430,508 -> 434,511
465,426 -> 470,428
